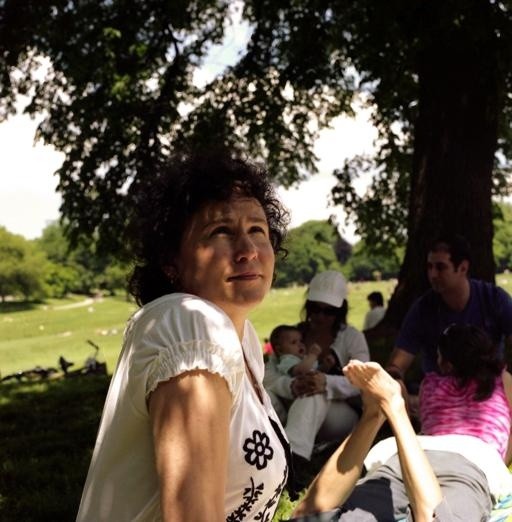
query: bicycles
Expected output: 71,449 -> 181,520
1,339 -> 107,387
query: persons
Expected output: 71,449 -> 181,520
74,145 -> 293,522
260,233 -> 511,522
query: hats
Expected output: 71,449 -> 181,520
308,270 -> 347,308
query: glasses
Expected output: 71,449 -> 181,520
311,303 -> 337,317
